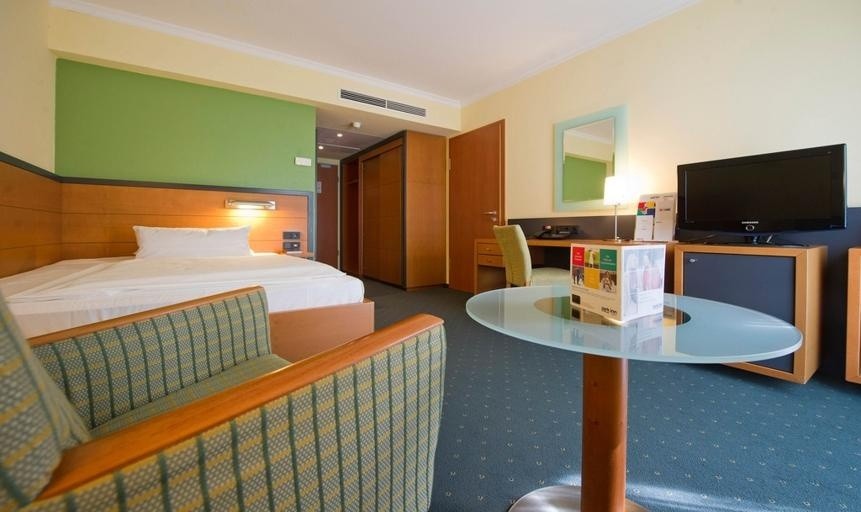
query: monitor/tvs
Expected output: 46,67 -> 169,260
677,144 -> 847,246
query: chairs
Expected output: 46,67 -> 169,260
493,224 -> 571,288
0,276 -> 445,512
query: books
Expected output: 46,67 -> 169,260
570,241 -> 668,322
636,192 -> 676,242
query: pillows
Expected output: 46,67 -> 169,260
127,222 -> 254,258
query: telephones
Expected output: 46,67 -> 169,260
534,226 -> 574,239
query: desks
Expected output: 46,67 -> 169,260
472,238 -> 638,299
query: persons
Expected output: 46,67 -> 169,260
577,271 -> 585,287
587,250 -> 599,267
600,270 -> 616,292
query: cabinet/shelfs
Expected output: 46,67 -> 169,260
340,131 -> 447,291
673,241 -> 829,385
845,246 -> 861,384
463,287 -> 800,511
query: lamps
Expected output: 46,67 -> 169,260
603,175 -> 628,240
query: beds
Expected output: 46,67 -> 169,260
1,227 -> 374,363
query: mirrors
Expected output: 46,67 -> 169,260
555,106 -> 628,212
